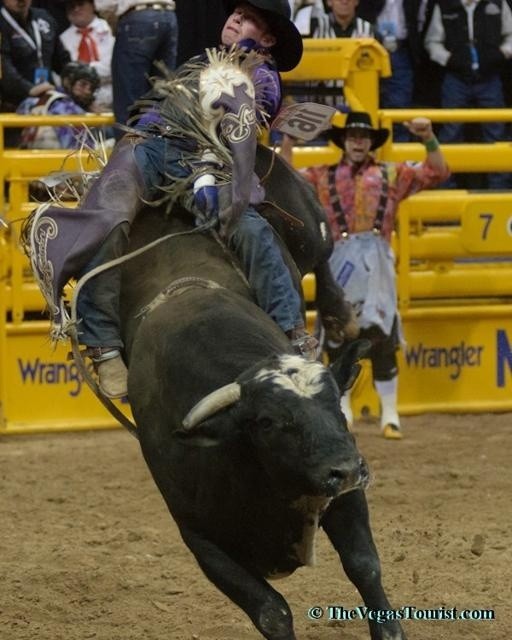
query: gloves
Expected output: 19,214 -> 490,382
192,184 -> 220,232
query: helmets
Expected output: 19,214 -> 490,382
60,61 -> 102,107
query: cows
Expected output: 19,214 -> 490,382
68,138 -> 409,640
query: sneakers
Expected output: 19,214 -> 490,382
87,342 -> 131,400
285,325 -> 320,364
381,420 -> 404,441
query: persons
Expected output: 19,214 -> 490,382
278,110 -> 453,439
22,0 -> 318,401
0,1 -> 512,191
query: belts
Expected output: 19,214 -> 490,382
118,2 -> 176,21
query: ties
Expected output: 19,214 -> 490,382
76,27 -> 100,64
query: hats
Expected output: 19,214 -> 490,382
231,1 -> 305,73
324,111 -> 391,152
